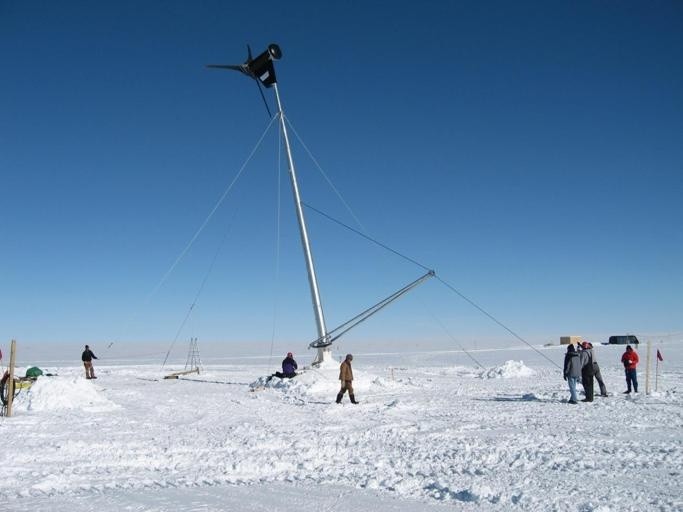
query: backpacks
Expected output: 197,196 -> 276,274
26,367 -> 42,377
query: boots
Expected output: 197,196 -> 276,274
350,395 -> 359,404
336,394 -> 343,404
86,376 -> 97,380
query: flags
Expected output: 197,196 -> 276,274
657,349 -> 664,362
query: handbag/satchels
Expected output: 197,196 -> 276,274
585,364 -> 593,376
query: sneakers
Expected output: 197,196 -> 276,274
569,394 -> 608,404
624,390 -> 631,393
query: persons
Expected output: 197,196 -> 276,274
563,340 -> 609,405
81,344 -> 98,380
620,345 -> 640,395
282,351 -> 298,378
335,353 -> 360,405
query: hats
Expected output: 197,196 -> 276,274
567,344 -> 575,351
346,354 -> 352,360
288,352 -> 292,358
626,346 -> 631,349
582,342 -> 593,349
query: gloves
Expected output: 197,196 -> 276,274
563,374 -> 568,381
629,359 -> 632,364
